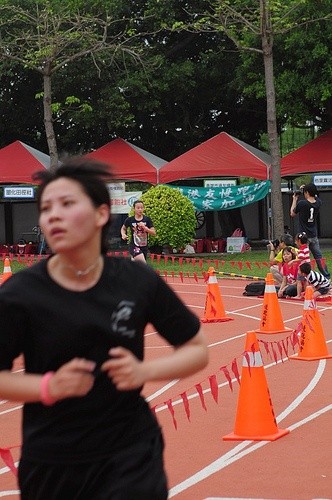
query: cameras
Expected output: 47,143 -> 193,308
286,188 -> 303,195
267,239 -> 279,249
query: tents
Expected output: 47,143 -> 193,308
269,128 -> 332,247
0,141 -> 65,186
75,137 -> 167,188
158,131 -> 271,260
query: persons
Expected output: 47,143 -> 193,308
266,231 -> 332,299
290,183 -> 331,283
121,200 -> 156,264
0,161 -> 211,500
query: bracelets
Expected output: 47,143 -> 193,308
40,372 -> 58,405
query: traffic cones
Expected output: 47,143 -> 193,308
221,331 -> 290,441
288,285 -> 332,361
254,272 -> 293,334
1,257 -> 12,284
200,266 -> 233,323
